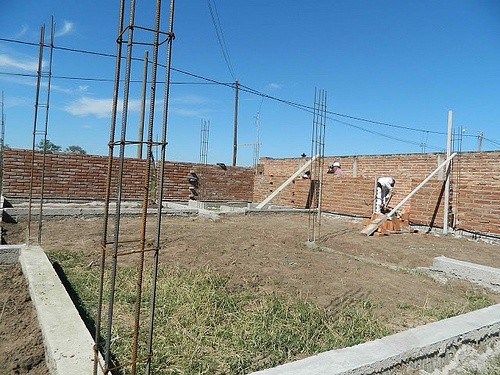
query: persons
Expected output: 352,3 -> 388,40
304,171 -> 311,179
333,162 -> 343,176
375,177 -> 396,196
